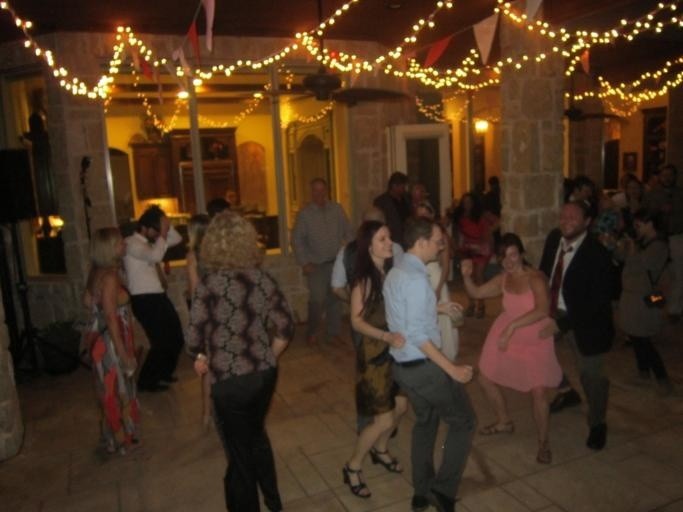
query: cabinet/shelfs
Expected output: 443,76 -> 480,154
126,140 -> 173,203
163,125 -> 241,217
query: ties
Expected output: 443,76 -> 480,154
551,246 -> 574,311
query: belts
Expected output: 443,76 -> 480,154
391,356 -> 432,367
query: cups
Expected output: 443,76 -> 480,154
451,308 -> 465,329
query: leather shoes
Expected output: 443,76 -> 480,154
135,374 -> 178,393
548,387 -> 583,414
586,421 -> 608,451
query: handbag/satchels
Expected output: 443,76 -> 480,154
644,290 -> 667,309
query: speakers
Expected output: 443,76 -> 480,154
0,148 -> 38,223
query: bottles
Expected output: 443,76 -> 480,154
225,186 -> 235,206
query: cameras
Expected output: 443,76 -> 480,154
618,226 -> 637,239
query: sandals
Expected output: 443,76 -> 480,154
464,302 -> 487,319
536,441 -> 554,463
480,419 -> 515,436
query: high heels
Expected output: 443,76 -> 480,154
370,446 -> 403,475
342,463 -> 372,500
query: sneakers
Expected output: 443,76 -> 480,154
411,489 -> 457,512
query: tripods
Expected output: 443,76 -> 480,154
0,222 -> 92,385
